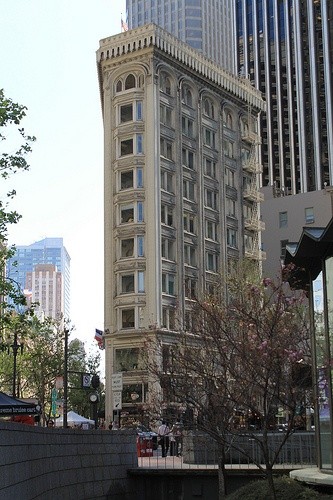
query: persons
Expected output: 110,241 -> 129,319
46,420 -> 53,427
158,421 -> 169,457
170,419 -> 182,456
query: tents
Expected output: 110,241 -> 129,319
50,411 -> 95,427
0,391 -> 42,417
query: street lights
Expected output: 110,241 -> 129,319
7,329 -> 24,398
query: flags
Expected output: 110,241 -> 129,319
95,329 -> 103,346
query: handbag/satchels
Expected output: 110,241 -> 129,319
158,438 -> 164,445
